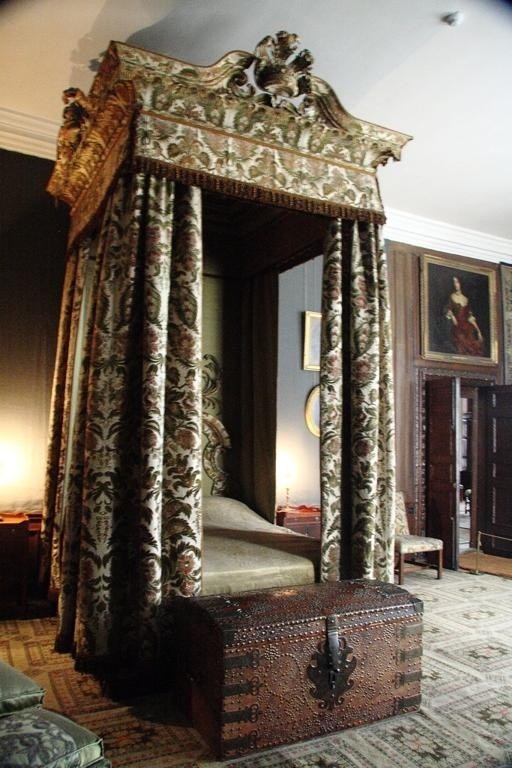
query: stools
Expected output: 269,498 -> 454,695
0,663 -> 114,767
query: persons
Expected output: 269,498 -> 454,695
443,273 -> 484,357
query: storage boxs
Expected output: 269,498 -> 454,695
178,580 -> 424,756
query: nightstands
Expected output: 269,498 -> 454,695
1,511 -> 29,608
278,505 -> 322,546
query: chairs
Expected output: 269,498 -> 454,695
393,490 -> 446,583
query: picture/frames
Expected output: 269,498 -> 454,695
415,253 -> 500,369
303,310 -> 323,437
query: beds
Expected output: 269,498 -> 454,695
190,354 -> 324,594
